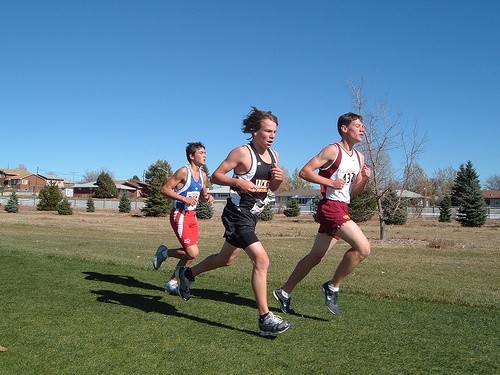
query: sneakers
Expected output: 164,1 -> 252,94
273,287 -> 291,314
258,311 -> 292,337
176,266 -> 195,301
153,245 -> 167,270
321,280 -> 340,314
165,280 -> 180,296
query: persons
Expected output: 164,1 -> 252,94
153,143 -> 214,294
273,113 -> 371,315
175,106 -> 290,336
